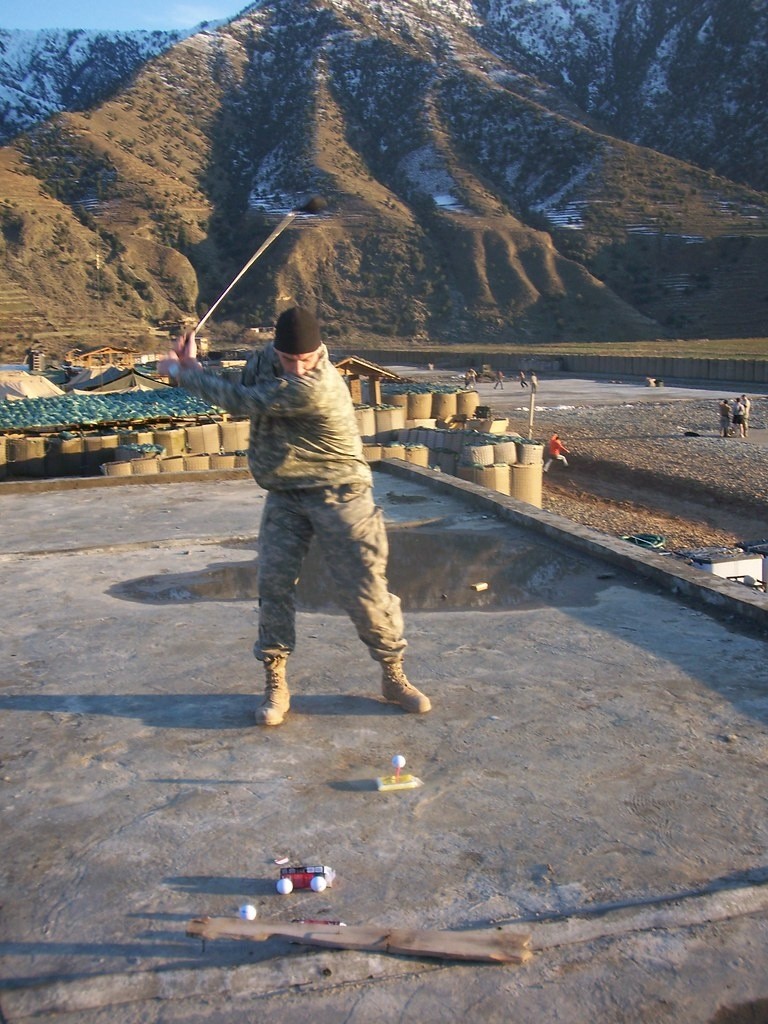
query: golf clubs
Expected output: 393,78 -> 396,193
185,194 -> 329,344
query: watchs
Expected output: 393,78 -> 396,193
169,362 -> 180,378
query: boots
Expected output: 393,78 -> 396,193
381,659 -> 431,713
255,659 -> 291,725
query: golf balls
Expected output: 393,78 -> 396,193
392,754 -> 406,768
310,876 -> 327,892
239,904 -> 257,920
276,878 -> 293,895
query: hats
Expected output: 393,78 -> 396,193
273,307 -> 322,354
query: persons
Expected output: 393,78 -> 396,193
543,434 -> 570,473
718,399 -> 732,437
520,371 -> 528,389
494,370 -> 504,390
465,368 -> 477,389
731,398 -> 745,438
531,372 -> 537,393
741,395 -> 750,437
156,307 -> 431,726
